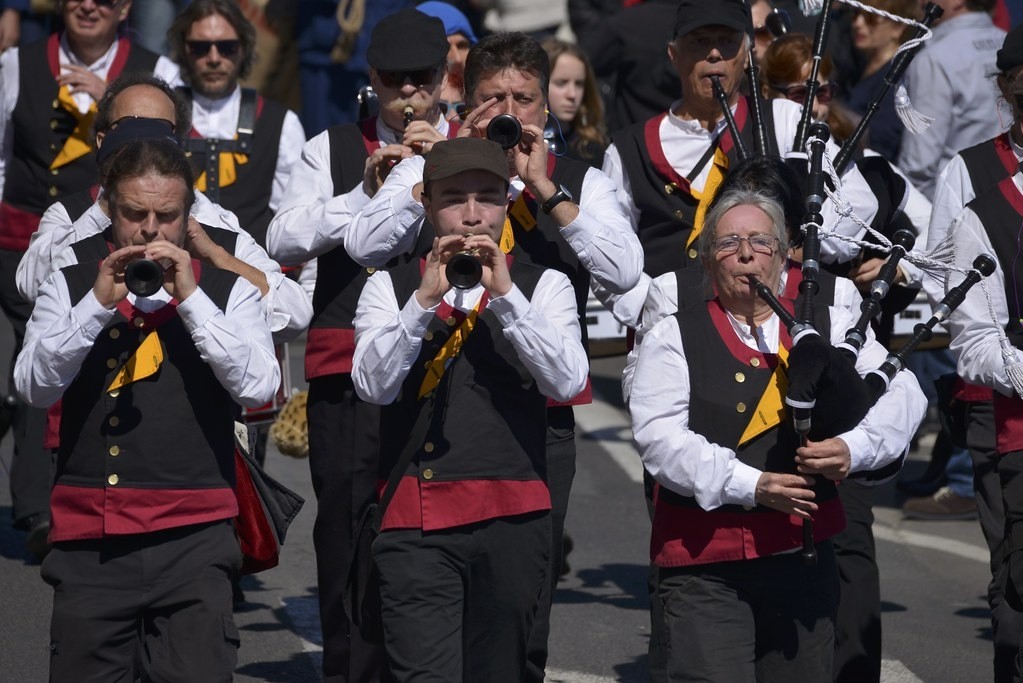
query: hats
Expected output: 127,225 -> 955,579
423,137 -> 510,192
367,7 -> 450,71
671,0 -> 750,40
416,0 -> 479,44
96,118 -> 182,166
996,20 -> 1023,70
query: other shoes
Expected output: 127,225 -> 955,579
25,512 -> 51,559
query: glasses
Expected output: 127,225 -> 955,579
94,0 -> 120,8
849,5 -> 883,24
771,80 -> 838,104
374,69 -> 443,88
184,39 -> 242,56
711,233 -> 783,251
104,116 -> 177,133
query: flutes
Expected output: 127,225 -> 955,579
710,0 -> 946,205
445,234 -> 483,290
124,252 -> 164,298
402,103 -> 416,161
485,114 -> 522,148
746,120 -> 998,565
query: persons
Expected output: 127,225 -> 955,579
1,0 -> 1023,683
350,137 -> 590,683
13,140 -> 281,683
630,190 -> 929,683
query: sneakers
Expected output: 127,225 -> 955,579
903,486 -> 977,519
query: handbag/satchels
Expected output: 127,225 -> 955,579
334,503 -> 385,642
